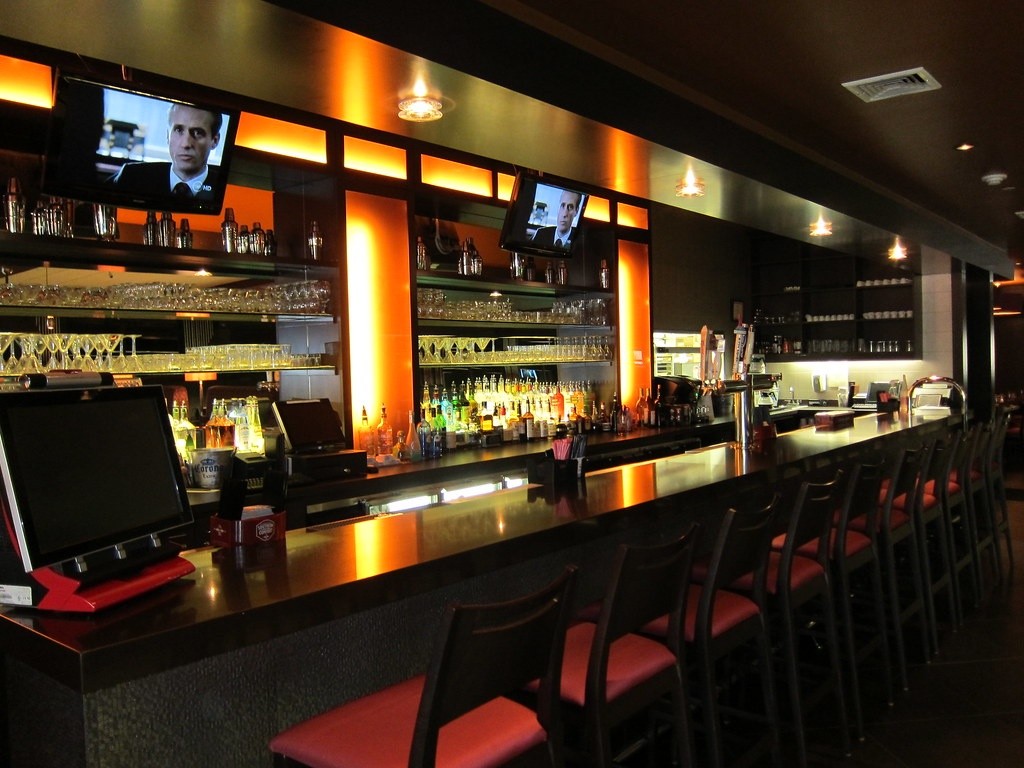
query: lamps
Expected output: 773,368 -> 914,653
888,251 -> 908,260
398,100 -> 444,123
809,227 -> 833,237
676,187 -> 705,200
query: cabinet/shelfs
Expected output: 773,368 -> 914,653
0,228 -> 344,379
417,273 -> 616,371
745,234 -> 919,362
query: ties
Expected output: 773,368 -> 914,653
555,239 -> 562,248
174,183 -> 190,203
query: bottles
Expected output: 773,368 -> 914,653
170,395 -> 266,489
143,211 -> 158,245
857,338 -> 911,352
94,204 -> 120,241
266,230 -> 275,254
46,196 -> 63,236
308,220 -> 322,263
64,198 -> 74,238
176,219 -> 192,249
250,222 -> 266,254
805,338 -> 855,353
158,210 -> 176,248
3,178 -> 25,234
31,201 -> 46,235
357,374 -> 665,465
417,237 -> 610,288
756,335 -> 802,355
237,225 -> 250,254
221,208 -> 238,252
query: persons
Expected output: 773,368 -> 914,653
526,189 -> 582,253
101,102 -> 223,208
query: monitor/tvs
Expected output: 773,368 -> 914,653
271,398 -> 347,452
44,73 -> 241,214
0,385 -> 193,574
498,171 -> 589,259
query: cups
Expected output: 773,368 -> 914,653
863,310 -> 913,319
187,447 -> 237,489
805,313 -> 854,321
413,289 -> 613,326
856,278 -> 909,286
144,343 -> 292,373
0,279 -> 258,315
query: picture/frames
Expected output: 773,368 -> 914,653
729,295 -> 744,323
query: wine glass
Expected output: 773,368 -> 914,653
0,333 -> 143,375
263,279 -> 331,314
416,335 -> 612,363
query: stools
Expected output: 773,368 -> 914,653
267,412 -> 1024,768
